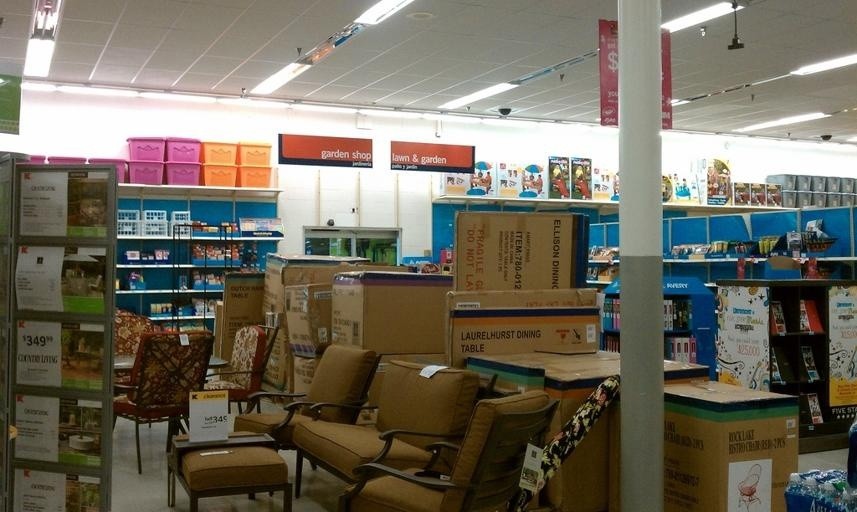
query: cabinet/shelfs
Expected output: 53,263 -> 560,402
702,278 -> 834,442
107,183 -> 284,355
584,206 -> 857,383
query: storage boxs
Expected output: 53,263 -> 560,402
764,170 -> 857,215
21,131 -> 278,192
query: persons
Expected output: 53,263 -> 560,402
551,162 -> 592,199
673,167 -> 780,209
445,169 -> 543,196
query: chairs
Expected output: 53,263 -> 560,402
108,328 -> 216,474
110,302 -> 167,358
160,426 -> 298,512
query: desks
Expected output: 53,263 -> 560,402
113,351 -> 139,376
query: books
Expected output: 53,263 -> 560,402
767,296 -> 828,426
599,294 -> 696,364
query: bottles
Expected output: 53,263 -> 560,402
759,234 -> 781,253
806,237 -> 839,253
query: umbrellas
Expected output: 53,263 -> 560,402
473,160 -> 493,172
525,163 -> 544,176
